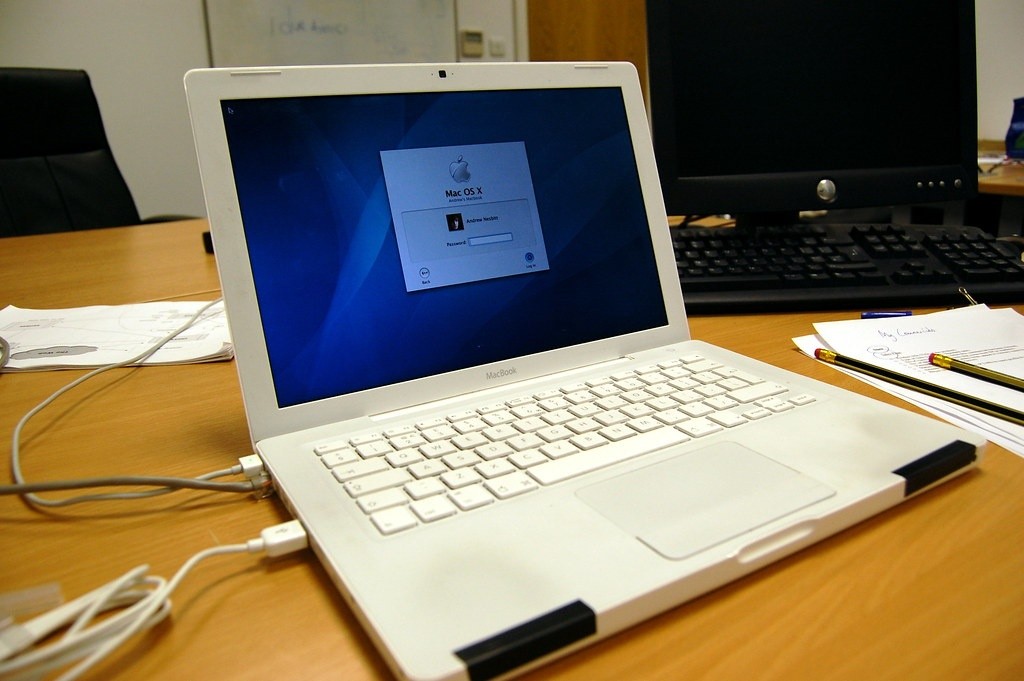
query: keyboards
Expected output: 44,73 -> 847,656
668,225 -> 1024,318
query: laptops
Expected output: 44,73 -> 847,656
183,61 -> 986,681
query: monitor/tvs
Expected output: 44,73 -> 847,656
642,0 -> 979,225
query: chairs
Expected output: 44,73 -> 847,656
0,68 -> 202,239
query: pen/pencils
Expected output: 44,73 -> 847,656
928,352 -> 1024,393
814,347 -> 1024,425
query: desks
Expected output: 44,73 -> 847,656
0,140 -> 1024,681
